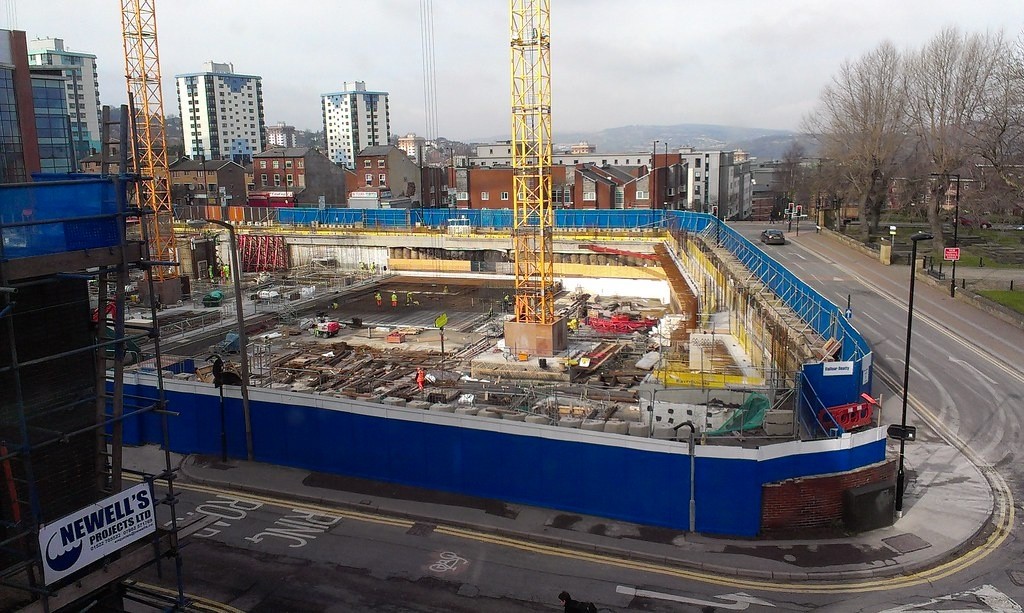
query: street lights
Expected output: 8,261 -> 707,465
887,232 -> 935,518
931,173 -> 960,298
664,143 -> 667,208
653,141 -> 659,207
187,218 -> 254,464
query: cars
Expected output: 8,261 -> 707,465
760,229 -> 786,245
952,214 -> 992,229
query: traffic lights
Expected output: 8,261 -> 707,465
712,206 -> 718,217
785,203 -> 794,215
793,206 -> 802,217
